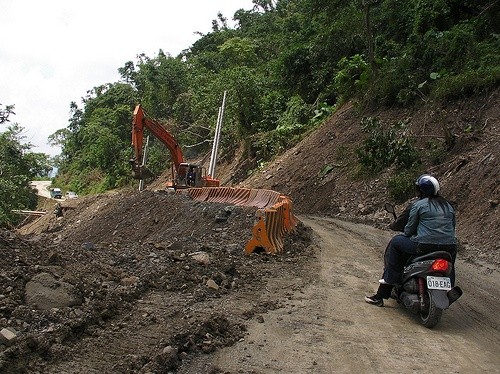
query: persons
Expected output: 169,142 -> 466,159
364,174 -> 458,307
183,167 -> 194,184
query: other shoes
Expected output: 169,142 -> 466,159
378,279 -> 389,284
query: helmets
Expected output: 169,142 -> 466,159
414,174 -> 439,195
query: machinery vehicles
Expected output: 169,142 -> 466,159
129,104 -> 220,188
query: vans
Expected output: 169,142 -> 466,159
65,192 -> 78,200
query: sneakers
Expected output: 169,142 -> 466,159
364,296 -> 383,306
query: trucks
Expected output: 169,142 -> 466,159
50,188 -> 62,199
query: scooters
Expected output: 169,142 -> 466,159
385,201 -> 463,328
